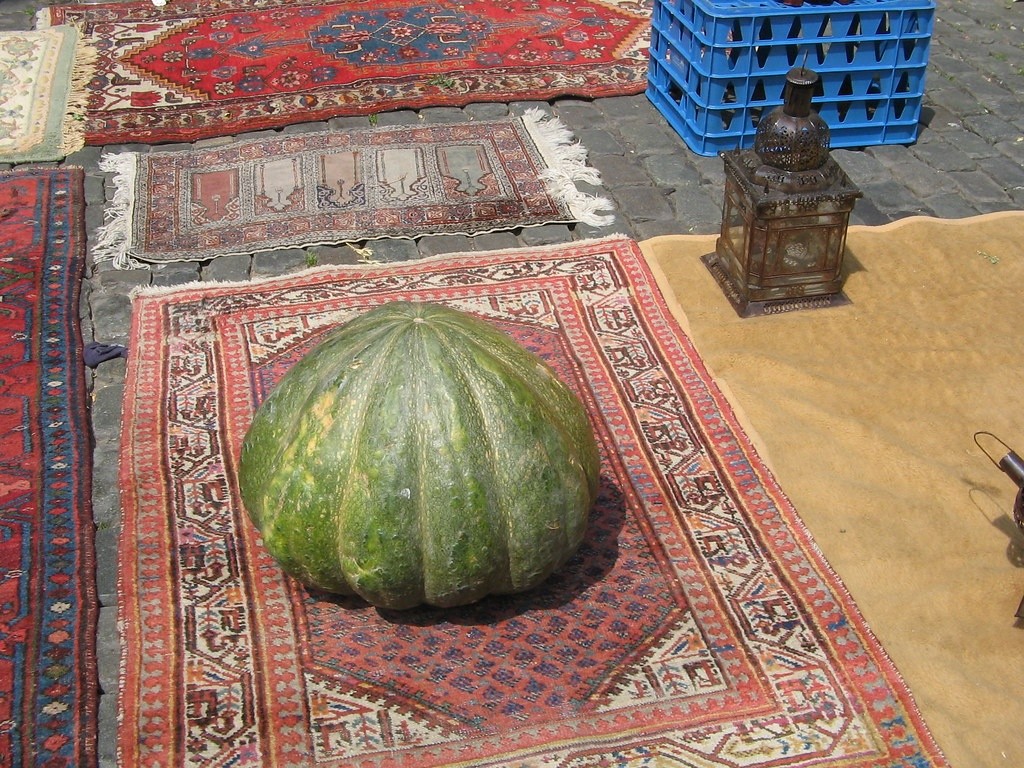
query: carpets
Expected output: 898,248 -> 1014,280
116,233 -> 952,767
636,211 -> 1024,767
37,0 -> 734,146
0,21 -> 101,164
92,104 -> 616,272
0,165 -> 107,768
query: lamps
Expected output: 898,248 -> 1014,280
697,47 -> 866,319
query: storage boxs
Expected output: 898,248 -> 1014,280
644,0 -> 937,157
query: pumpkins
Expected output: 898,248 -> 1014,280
237,301 -> 604,610
